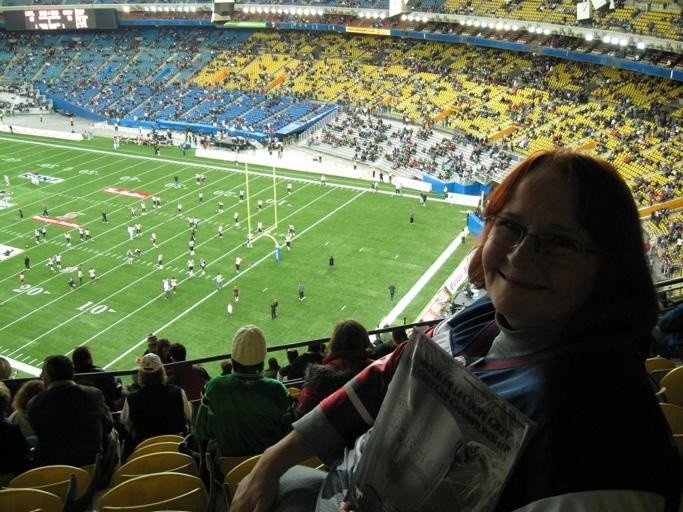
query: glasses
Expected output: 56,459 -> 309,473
484,214 -> 600,265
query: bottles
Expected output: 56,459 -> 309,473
23,445 -> 40,470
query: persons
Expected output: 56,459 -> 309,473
230,148 -> 683,511
1,301 -> 682,487
2,0 -> 683,319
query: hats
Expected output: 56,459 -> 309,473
141,353 -> 161,373
232,325 -> 266,366
